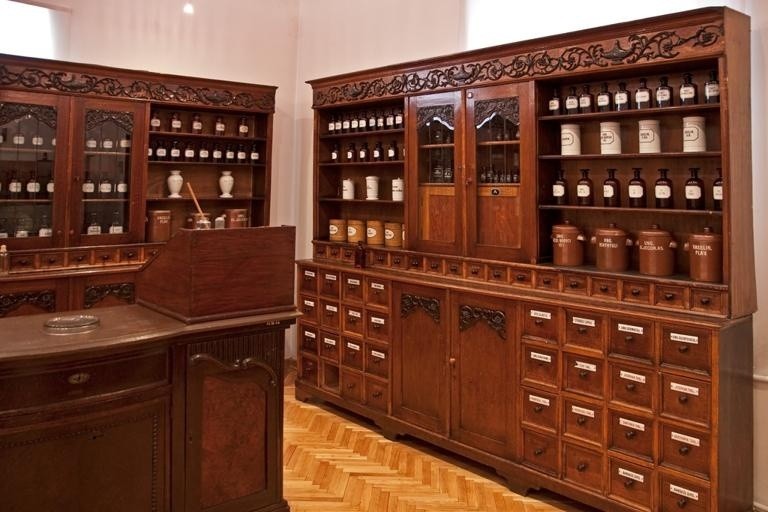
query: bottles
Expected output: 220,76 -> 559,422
215,216 -> 225,229
148,107 -> 261,163
477,122 -> 520,182
0,121 -> 131,238
549,167 -> 723,212
355,240 -> 366,269
429,128 -> 454,182
328,107 -> 406,162
196,217 -> 211,230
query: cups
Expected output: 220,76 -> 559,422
341,176 -> 406,201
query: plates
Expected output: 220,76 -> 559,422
548,70 -> 717,118
42,314 -> 99,334
